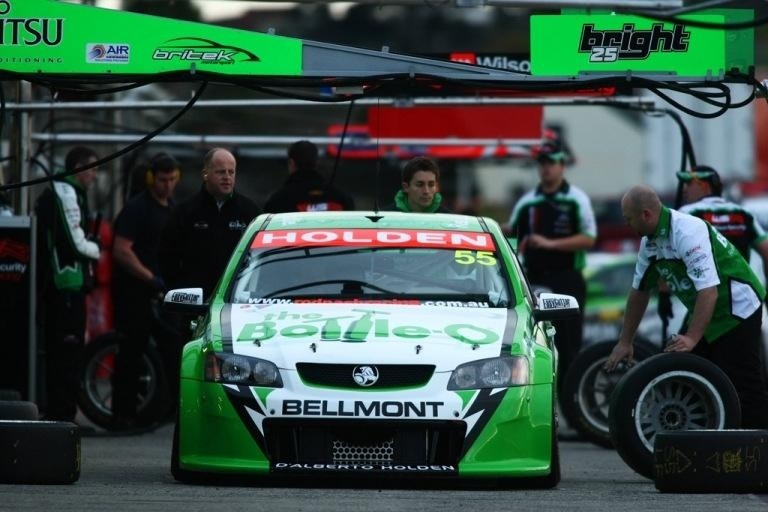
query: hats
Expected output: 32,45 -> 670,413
676,166 -> 720,188
534,142 -> 566,162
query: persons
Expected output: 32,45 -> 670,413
604,166 -> 768,430
391,157 -> 454,213
37,143 -> 256,430
264,140 -> 355,213
505,140 -> 598,430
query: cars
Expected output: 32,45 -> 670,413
579,248 -> 689,353
159,208 -> 582,486
